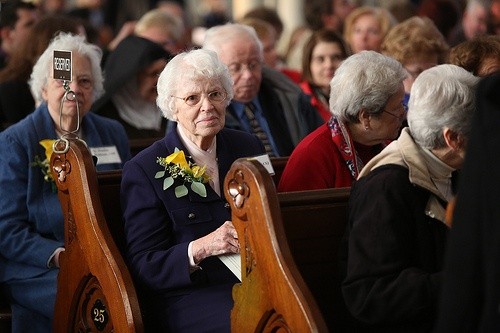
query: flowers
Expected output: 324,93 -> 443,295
155,146 -> 210,199
32,139 -> 61,193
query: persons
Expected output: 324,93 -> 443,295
0,0 -> 499,332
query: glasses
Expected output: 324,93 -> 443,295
383,104 -> 408,123
140,71 -> 159,80
171,90 -> 228,106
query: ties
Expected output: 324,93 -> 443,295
244,103 -> 276,157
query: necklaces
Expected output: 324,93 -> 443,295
427,165 -> 450,198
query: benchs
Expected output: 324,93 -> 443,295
224,159 -> 375,333
50,138 -> 288,333
